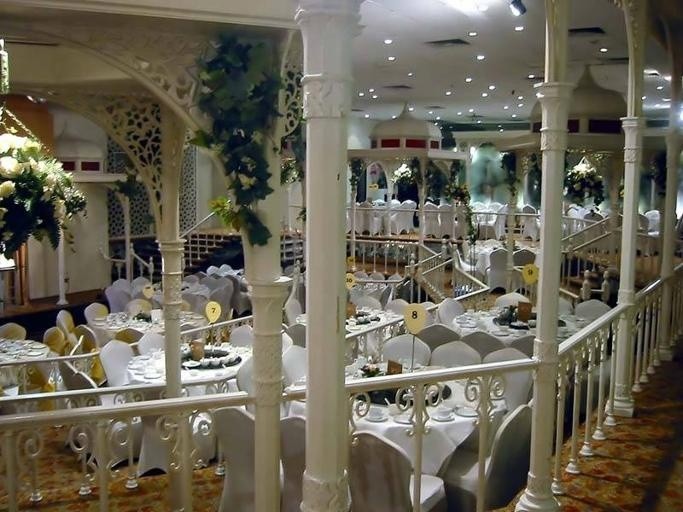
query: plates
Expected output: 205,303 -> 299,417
91,307 -> 203,332
458,305 -> 584,340
128,339 -> 253,381
293,375 -> 488,424
0,336 -> 47,362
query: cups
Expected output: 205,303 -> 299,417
346,306 -> 398,334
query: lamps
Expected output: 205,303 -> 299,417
509,0 -> 526,17
